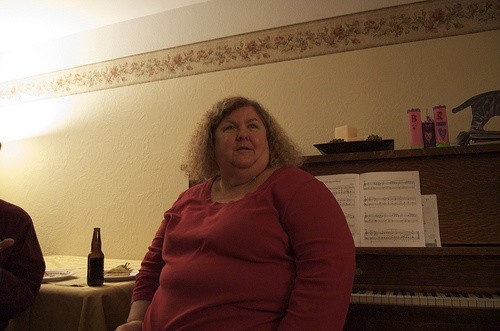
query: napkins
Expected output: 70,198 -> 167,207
48,279 -> 87,287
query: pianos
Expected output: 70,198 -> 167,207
189,140 -> 500,331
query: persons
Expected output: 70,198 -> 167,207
0,199 -> 46,331
116,96 -> 355,331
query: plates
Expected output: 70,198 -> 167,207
40,270 -> 74,282
103,270 -> 139,282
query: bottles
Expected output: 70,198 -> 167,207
87,228 -> 104,287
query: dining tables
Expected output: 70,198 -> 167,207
4,255 -> 142,331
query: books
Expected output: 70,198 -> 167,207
314,171 -> 441,248
